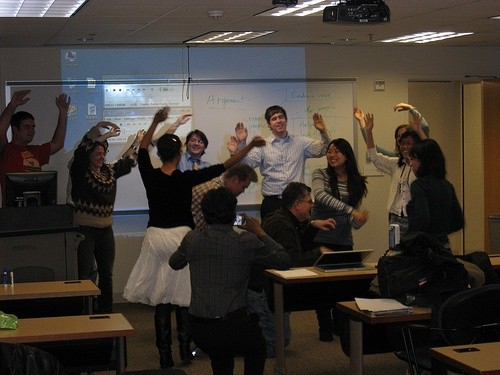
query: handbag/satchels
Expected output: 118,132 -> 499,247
376,234 -> 497,307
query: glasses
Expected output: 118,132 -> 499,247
190,138 -> 204,144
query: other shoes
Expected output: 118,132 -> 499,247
319,327 -> 331,342
333,308 -> 342,335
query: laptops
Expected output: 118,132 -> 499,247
313,249 -> 376,272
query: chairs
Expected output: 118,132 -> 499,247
0,342 -> 67,375
396,283 -> 500,375
13,266 -> 55,283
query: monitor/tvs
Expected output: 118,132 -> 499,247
0,171 -> 56,207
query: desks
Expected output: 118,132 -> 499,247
265,254 -> 500,375
0,313 -> 135,375
337,301 -> 432,375
430,341 -> 500,375
0,280 -> 101,315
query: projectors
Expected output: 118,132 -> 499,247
323,3 -> 391,25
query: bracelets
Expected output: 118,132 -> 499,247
131,145 -> 137,150
311,220 -> 314,223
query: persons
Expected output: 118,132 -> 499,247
70,122 -> 147,313
0,89 -> 71,208
246,182 -> 361,356
168,187 -> 291,375
164,113 -> 238,173
236,106 -> 329,216
123,107 -> 266,366
406,139 -> 465,254
312,138 -> 369,331
352,103 -> 430,248
191,164 -> 258,232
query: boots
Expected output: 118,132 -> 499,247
176,305 -> 193,362
154,308 -> 173,369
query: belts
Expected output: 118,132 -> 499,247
216,308 -> 248,319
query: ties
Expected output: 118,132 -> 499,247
193,160 -> 197,172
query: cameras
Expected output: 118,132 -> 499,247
234,213 -> 245,225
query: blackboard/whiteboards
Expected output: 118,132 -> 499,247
5,78 -> 359,214
